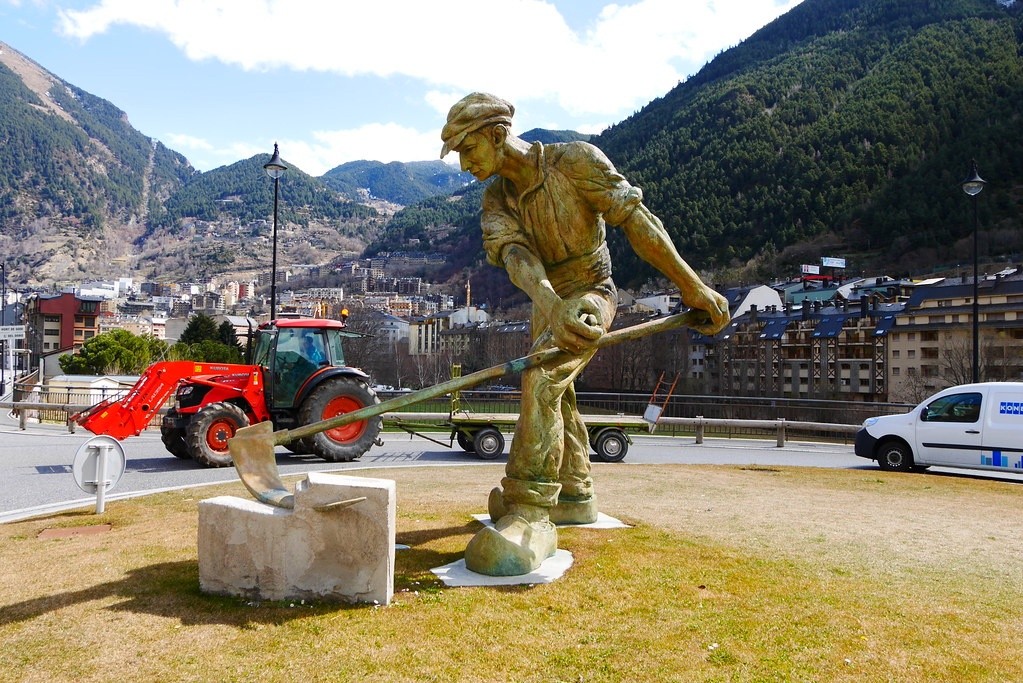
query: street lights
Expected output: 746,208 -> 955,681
959,161 -> 990,384
263,142 -> 289,325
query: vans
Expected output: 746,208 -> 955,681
855,380 -> 1022,477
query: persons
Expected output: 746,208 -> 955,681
440,92 -> 731,577
301,337 -> 323,361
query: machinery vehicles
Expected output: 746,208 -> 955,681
67,308 -> 385,469
